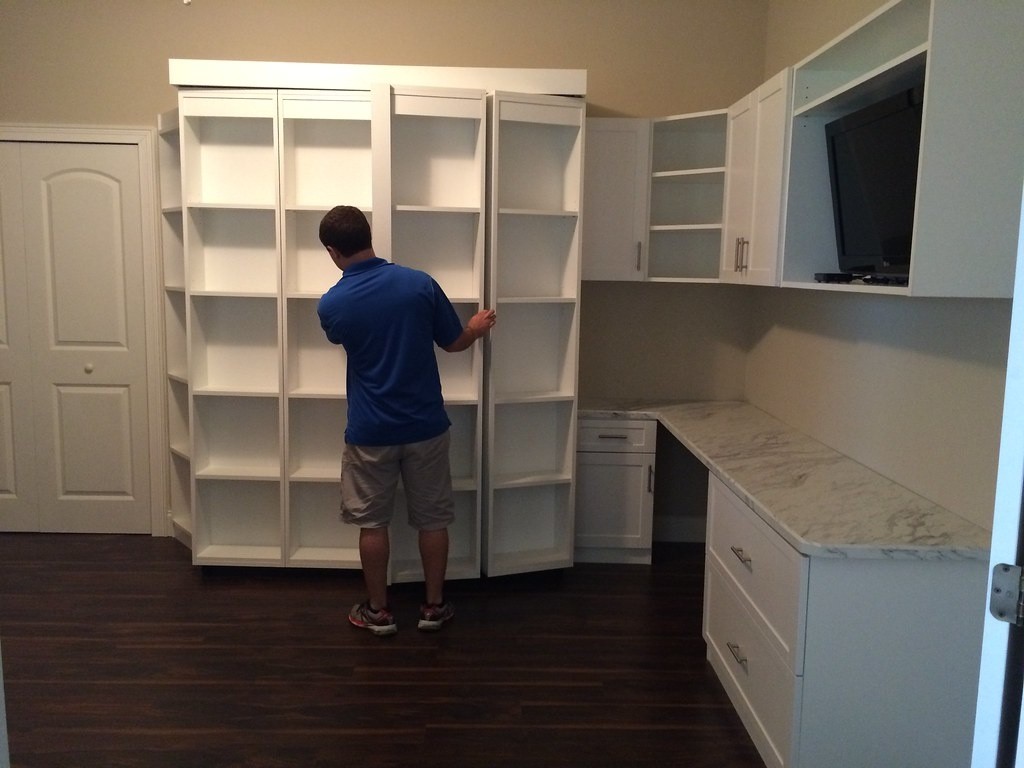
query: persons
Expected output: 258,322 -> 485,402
316,206 -> 496,635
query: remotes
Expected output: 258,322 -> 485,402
814,272 -> 909,287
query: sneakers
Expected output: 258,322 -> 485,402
418,600 -> 453,629
348,598 -> 398,635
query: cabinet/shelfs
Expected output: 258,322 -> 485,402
157,0 -> 1023,768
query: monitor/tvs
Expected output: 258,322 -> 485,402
824,84 -> 925,273
